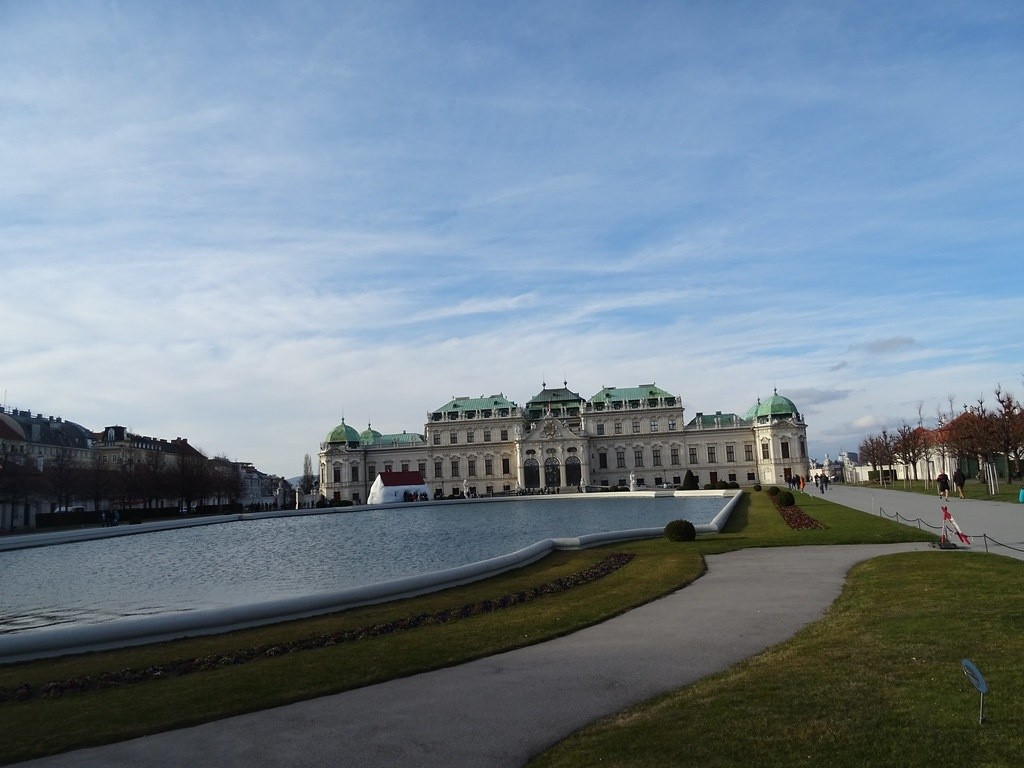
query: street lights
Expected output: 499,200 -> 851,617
663,469 -> 667,484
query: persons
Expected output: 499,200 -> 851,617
262,502 -> 280,510
809,473 -> 831,494
953,468 -> 966,499
516,486 -> 560,495
937,469 -> 950,502
403,490 -> 428,502
101,509 -> 119,527
787,475 -> 805,494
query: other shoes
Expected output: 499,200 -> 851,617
945,499 -> 949,502
959,497 -> 965,499
940,495 -> 942,499
